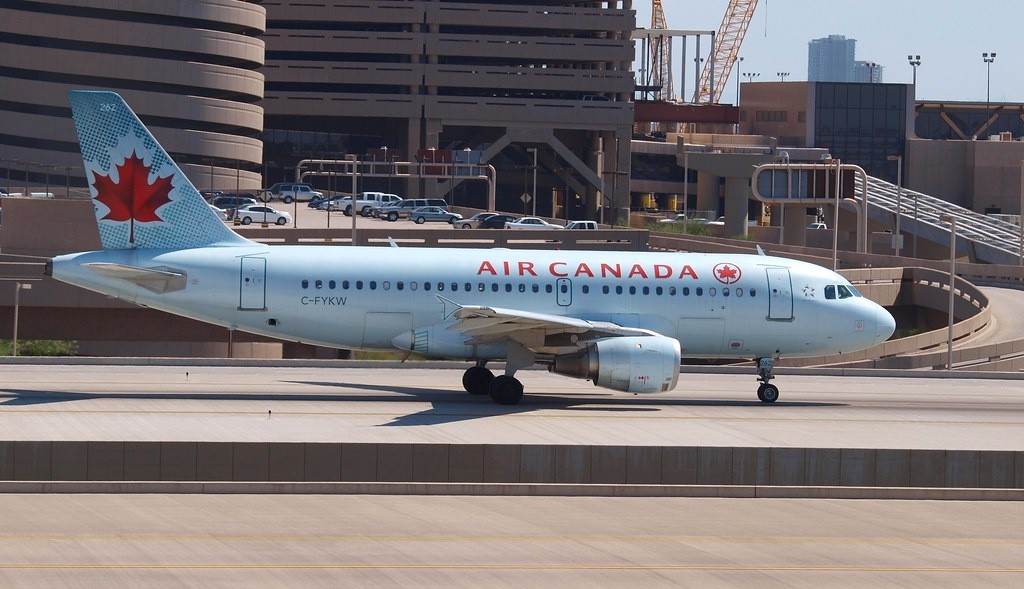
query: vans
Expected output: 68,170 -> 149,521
564,221 -> 598,230
263,183 -> 314,199
378,199 -> 448,222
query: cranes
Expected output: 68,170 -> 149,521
650,0 -> 759,133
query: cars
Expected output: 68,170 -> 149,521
196,190 -> 292,225
407,206 -> 564,230
656,214 -> 828,229
308,195 -> 351,212
365,202 -> 394,218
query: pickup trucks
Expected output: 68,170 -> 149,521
334,192 -> 403,217
278,185 -> 324,204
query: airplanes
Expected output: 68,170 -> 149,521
44,91 -> 898,405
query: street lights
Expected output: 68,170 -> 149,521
982,52 -> 996,140
742,73 -> 790,82
908,55 -> 921,84
866,62 -> 876,83
736,57 -> 744,107
